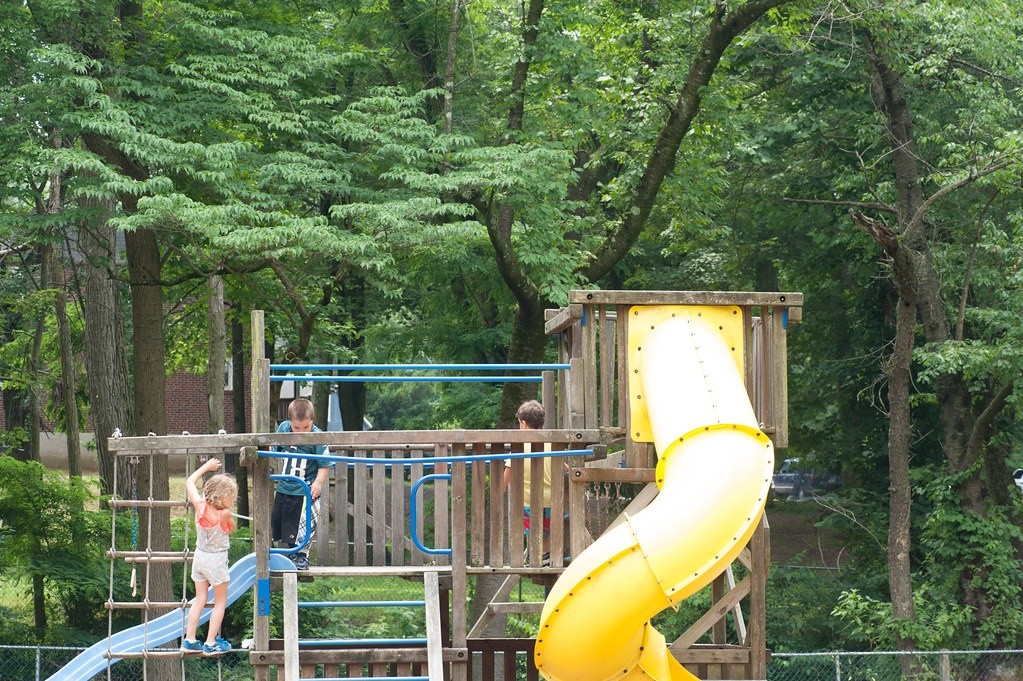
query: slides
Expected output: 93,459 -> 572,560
533,304 -> 774,681
39,552 -> 296,681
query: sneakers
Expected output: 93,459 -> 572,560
203,636 -> 231,656
293,556 -> 310,570
288,545 -> 298,560
180,639 -> 204,653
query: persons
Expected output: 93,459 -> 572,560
179,458 -> 238,655
270,399 -> 336,571
502,399 -> 570,566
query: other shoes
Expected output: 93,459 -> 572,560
523,552 -> 551,567
